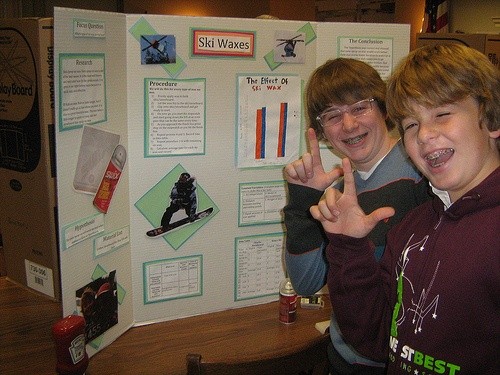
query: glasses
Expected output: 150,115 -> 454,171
316,98 -> 376,127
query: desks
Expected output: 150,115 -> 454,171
1,294 -> 334,375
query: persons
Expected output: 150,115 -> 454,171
282,55 -> 424,375
308,40 -> 500,375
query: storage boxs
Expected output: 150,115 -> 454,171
0,16 -> 61,299
415,33 -> 500,71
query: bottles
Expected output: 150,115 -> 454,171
279,271 -> 297,325
